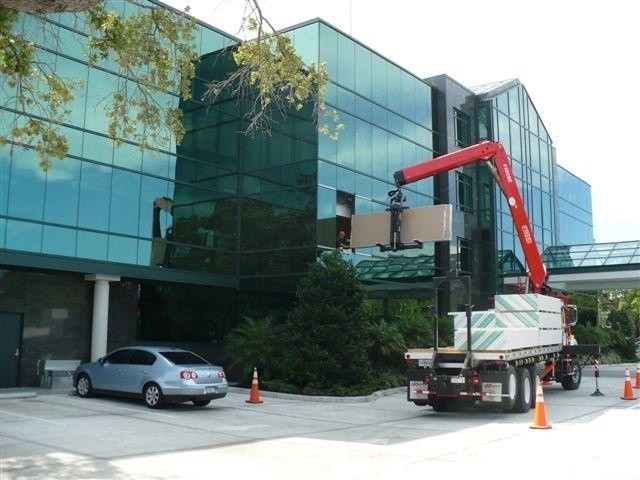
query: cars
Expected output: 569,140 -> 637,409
72,346 -> 229,409
634,341 -> 640,360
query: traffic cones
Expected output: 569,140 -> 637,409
530,380 -> 553,430
632,360 -> 640,389
620,368 -> 638,400
245,367 -> 264,404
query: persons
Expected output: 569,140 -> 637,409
337,231 -> 351,250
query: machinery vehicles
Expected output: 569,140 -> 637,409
373,139 -> 605,416
